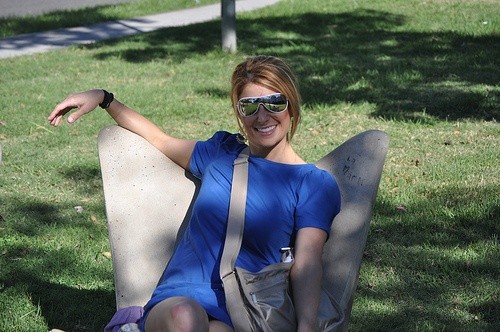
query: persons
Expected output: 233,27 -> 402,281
49,56 -> 342,332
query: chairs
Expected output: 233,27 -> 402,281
98,124 -> 391,332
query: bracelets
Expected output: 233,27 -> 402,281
99,89 -> 114,109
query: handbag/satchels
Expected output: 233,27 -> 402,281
222,248 -> 345,332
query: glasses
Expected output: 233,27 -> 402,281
237,93 -> 288,117
